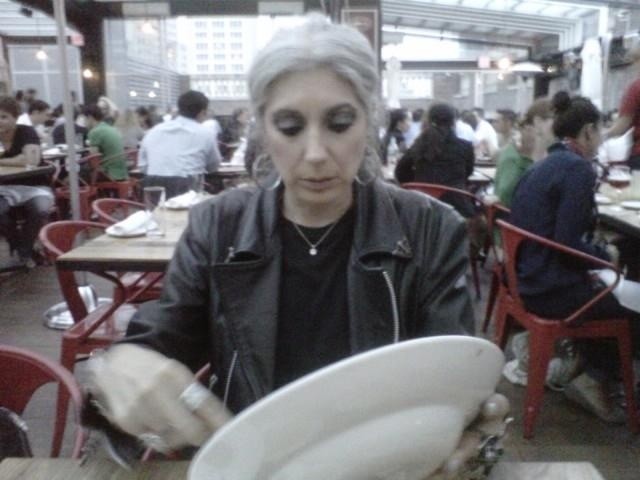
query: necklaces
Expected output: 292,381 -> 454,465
285,202 -> 354,257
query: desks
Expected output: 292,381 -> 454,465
0,166 -> 55,182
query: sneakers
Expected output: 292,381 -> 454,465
563,373 -> 627,427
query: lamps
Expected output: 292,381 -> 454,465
507,60 -> 544,77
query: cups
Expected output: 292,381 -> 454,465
143,187 -> 167,237
608,165 -> 632,194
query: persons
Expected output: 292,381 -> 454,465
394,102 -> 483,301
74,12 -> 510,479
140,89 -> 223,199
492,107 -> 522,152
507,90 -> 639,425
379,108 -> 412,178
17,99 -> 51,133
80,103 -> 129,200
604,75 -> 640,170
490,97 -> 549,282
459,111 -> 497,158
0,94 -> 57,276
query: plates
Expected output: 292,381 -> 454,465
187,336 -> 505,480
106,221 -> 157,237
620,200 -> 640,210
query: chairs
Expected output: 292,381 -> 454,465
91,197 -> 147,223
48,152 -> 103,238
38,221 -> 167,457
400,183 -> 484,298
90,150 -> 140,219
0,345 -> 85,458
495,219 -> 639,438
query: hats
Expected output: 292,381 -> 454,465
528,97 -> 558,119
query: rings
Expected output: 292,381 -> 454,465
471,427 -> 504,478
177,381 -> 211,414
134,431 -> 174,462
